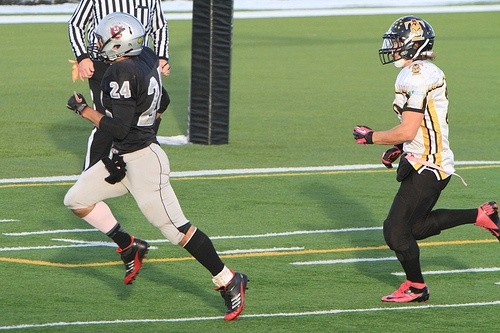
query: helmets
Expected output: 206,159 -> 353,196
93,12 -> 145,61
378,15 -> 435,65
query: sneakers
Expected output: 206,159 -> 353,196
381,279 -> 430,303
214,268 -> 249,320
473,200 -> 500,242
116,235 -> 150,285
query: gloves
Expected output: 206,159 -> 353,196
67,90 -> 88,114
381,145 -> 403,168
352,125 -> 374,144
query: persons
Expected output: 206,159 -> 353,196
67,0 -> 171,172
353,16 -> 500,303
63,12 -> 250,321
